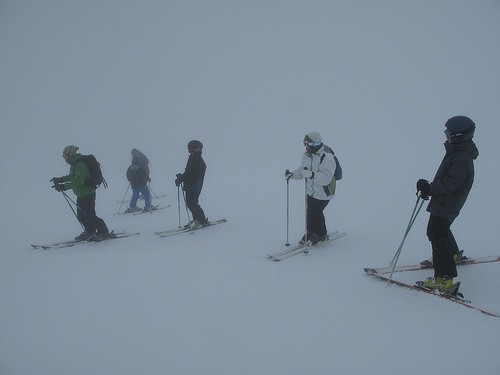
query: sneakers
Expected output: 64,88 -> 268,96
453,253 -> 461,264
424,275 -> 453,291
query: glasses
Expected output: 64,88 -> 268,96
444,131 -> 450,141
303,141 -> 308,150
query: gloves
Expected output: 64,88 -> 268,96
417,179 -> 429,199
50,177 -> 63,183
302,170 -> 312,178
284,172 -> 293,180
175,179 -> 181,186
176,174 -> 183,182
52,184 -> 65,192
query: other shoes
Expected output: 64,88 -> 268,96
189,220 -> 207,228
310,233 -> 325,245
186,219 -> 195,227
302,232 -> 312,242
87,231 -> 109,241
75,231 -> 96,241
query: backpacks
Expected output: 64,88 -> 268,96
73,154 -> 102,186
319,154 -> 343,180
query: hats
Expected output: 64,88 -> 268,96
305,132 -> 322,143
63,145 -> 79,157
188,140 -> 203,153
445,116 -> 475,143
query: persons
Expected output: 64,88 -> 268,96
415,116 -> 479,291
285,131 -> 336,246
175,140 -> 208,230
50,145 -> 109,242
126,149 -> 156,211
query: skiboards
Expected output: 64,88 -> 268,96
262,229 -> 346,263
115,193 -> 167,204
29,227 -> 142,250
154,216 -> 228,238
112,206 -> 171,218
363,256 -> 500,319
